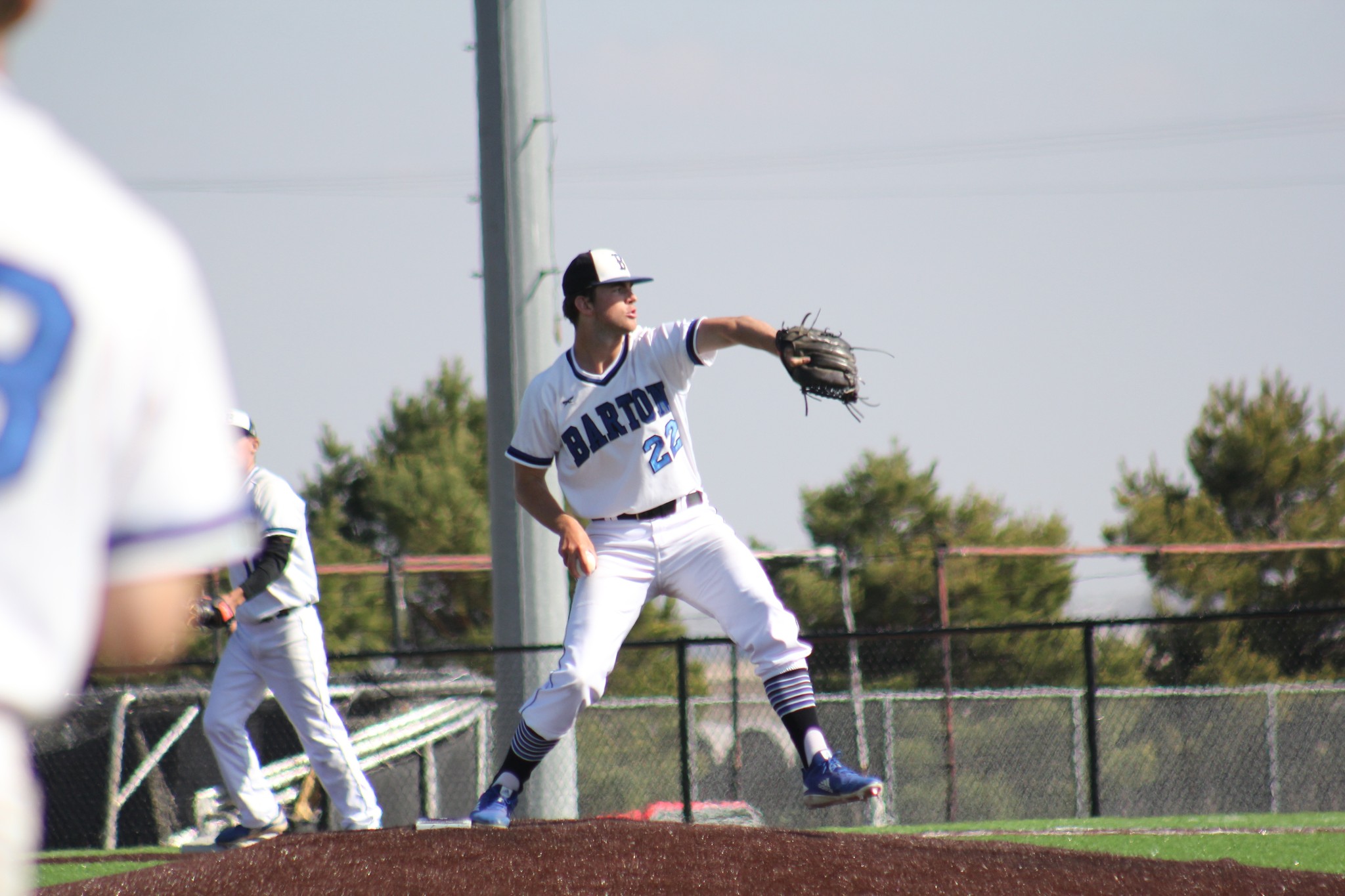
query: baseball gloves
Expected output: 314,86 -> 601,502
183,593 -> 236,629
774,326 -> 859,404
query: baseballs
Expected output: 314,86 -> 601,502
574,550 -> 597,576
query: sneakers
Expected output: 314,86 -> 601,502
469,784 -> 518,829
214,805 -> 289,849
800,751 -> 884,808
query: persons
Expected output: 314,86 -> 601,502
189,410 -> 382,850
468,249 -> 884,830
0,1 -> 269,896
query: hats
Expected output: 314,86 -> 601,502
562,249 -> 653,296
230,411 -> 257,436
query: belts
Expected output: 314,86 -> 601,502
591,492 -> 702,523
255,608 -> 292,624
